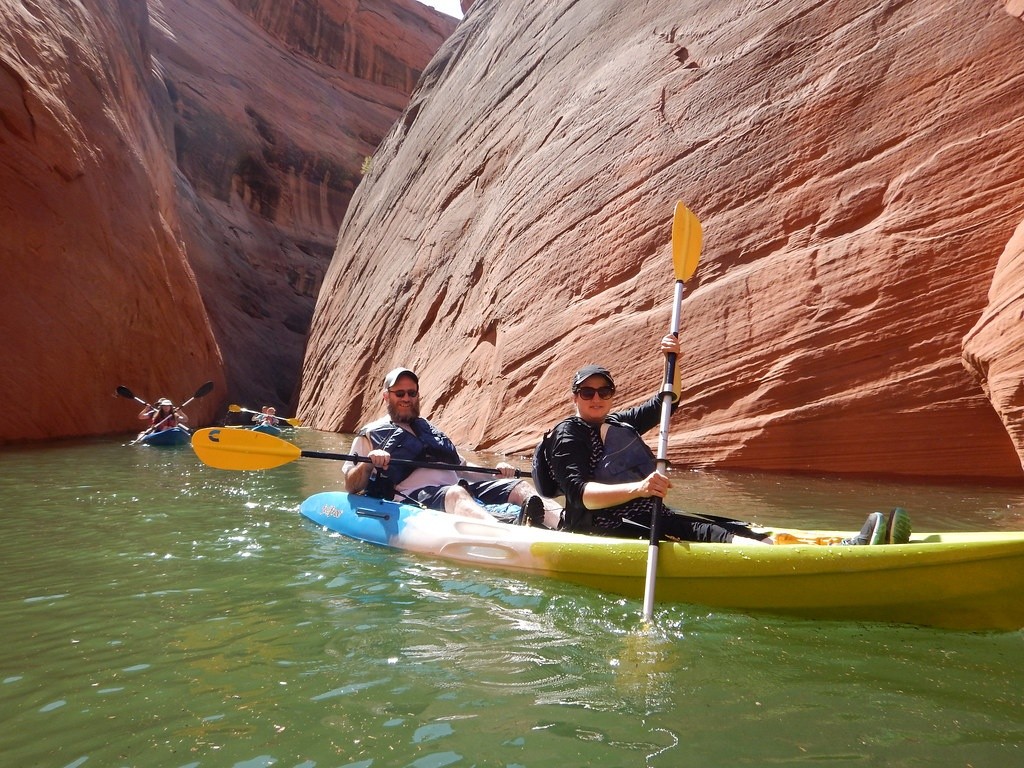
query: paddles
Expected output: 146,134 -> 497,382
640,196 -> 706,623
189,426 -> 533,481
228,404 -> 301,427
115,384 -> 192,433
130,380 -> 215,446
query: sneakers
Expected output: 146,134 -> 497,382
884,508 -> 910,544
843,511 -> 886,546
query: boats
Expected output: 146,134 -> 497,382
300,492 -> 1024,632
137,426 -> 190,446
252,424 -> 281,437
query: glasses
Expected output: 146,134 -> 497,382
389,389 -> 419,397
577,385 -> 614,400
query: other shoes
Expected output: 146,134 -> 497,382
519,496 -> 545,528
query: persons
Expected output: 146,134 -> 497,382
340,367 -> 569,532
139,397 -> 189,434
531,331 -> 911,544
251,405 -> 279,427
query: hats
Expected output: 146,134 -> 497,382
267,408 -> 275,412
154,398 -> 166,405
384,368 -> 422,389
160,400 -> 174,406
572,365 -> 614,392
